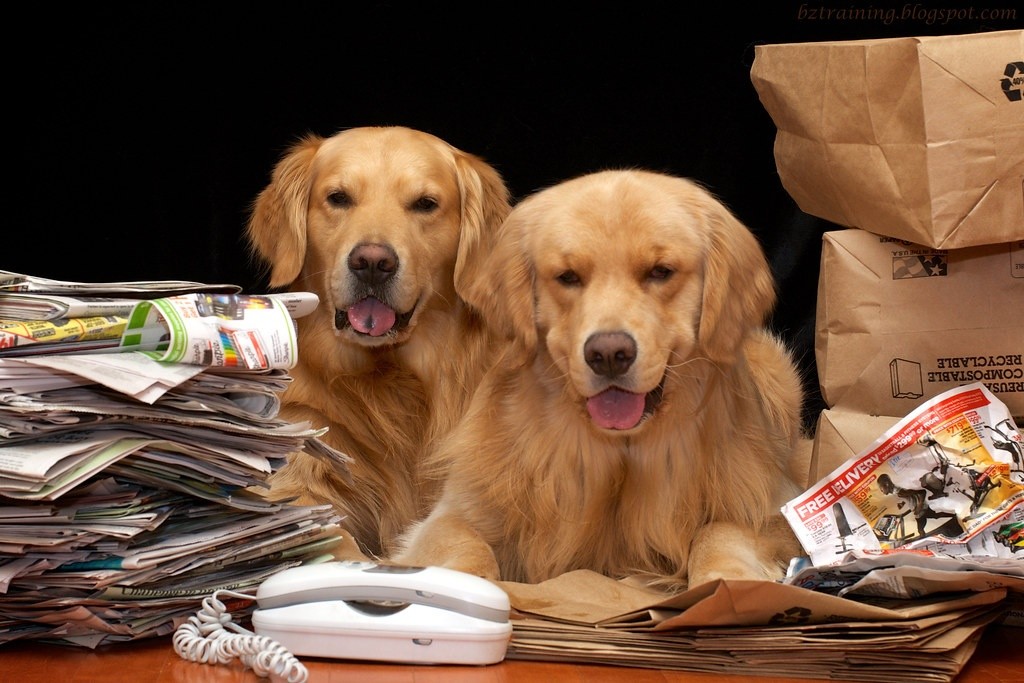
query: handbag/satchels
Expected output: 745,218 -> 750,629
750,30 -> 1024,250
491,568 -> 1014,683
815,230 -> 1024,419
807,409 -> 905,489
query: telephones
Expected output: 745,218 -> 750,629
251,559 -> 514,666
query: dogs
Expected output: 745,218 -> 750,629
386,170 -> 815,589
244,126 -> 516,563
